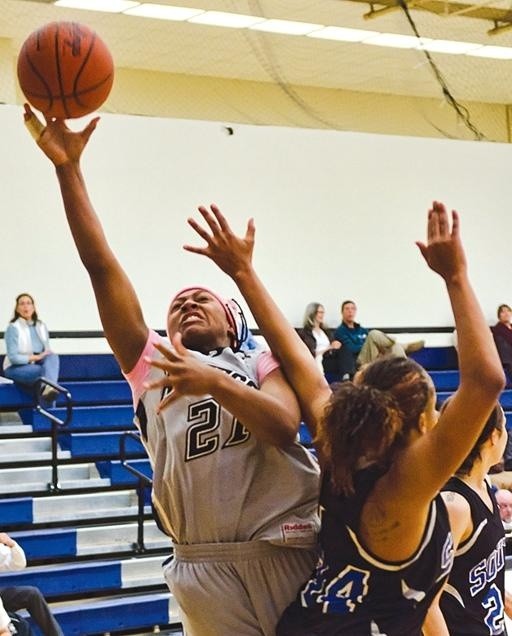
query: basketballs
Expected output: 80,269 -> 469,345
17,22 -> 114,120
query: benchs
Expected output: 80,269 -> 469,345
0,499 -> 171,636
1,352 -> 156,508
410,346 -> 511,435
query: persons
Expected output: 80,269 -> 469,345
4,293 -> 60,408
0,531 -> 63,636
23,102 -> 324,636
